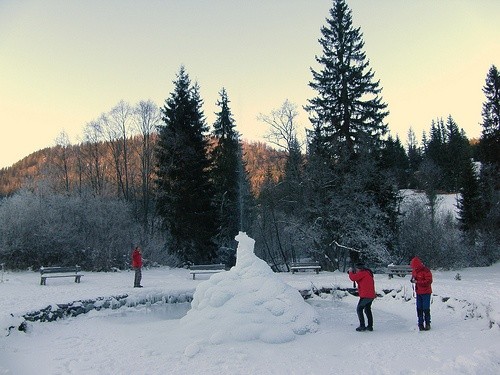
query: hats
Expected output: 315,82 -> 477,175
410,257 -> 424,272
354,262 -> 365,269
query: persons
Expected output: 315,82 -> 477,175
349,262 -> 377,332
410,256 -> 433,330
132,247 -> 143,287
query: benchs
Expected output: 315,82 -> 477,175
190,262 -> 225,280
40,264 -> 84,285
385,264 -> 412,279
291,261 -> 321,274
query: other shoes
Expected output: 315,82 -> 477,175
365,325 -> 373,331
426,322 -> 430,330
419,324 -> 425,331
356,325 -> 366,331
